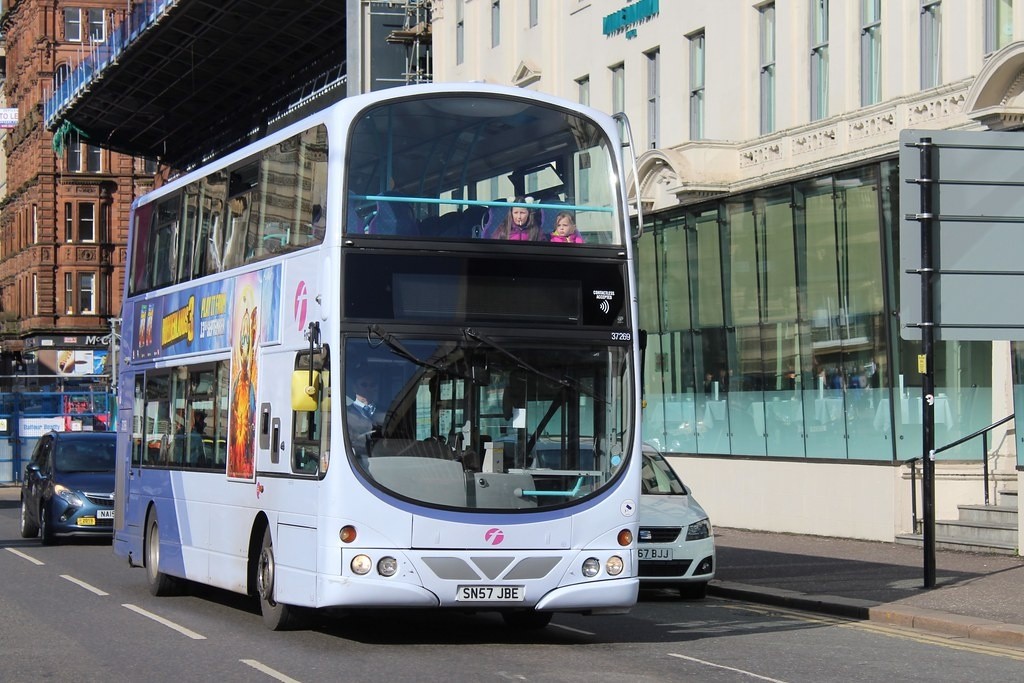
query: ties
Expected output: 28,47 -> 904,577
363,405 -> 372,418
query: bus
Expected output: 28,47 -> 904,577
107,80 -> 650,633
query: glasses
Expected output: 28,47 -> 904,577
357,382 -> 380,388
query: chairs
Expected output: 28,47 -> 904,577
346,198 -> 577,237
132,437 -> 226,470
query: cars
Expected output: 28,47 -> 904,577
19,429 -> 151,547
493,435 -> 717,601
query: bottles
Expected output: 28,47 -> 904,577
322,450 -> 330,472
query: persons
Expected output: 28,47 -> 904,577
56,446 -> 86,471
703,362 -> 883,426
346,371 -> 409,457
490,196 -> 548,241
550,211 -> 584,243
